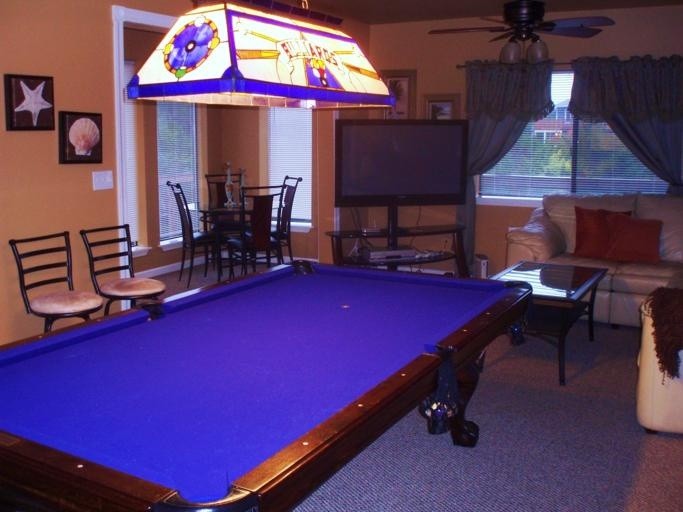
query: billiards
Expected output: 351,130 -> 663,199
508,321 -> 525,347
420,394 -> 479,448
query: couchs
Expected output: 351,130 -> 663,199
503,190 -> 683,333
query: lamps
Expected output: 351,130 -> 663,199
497,26 -> 550,66
127,2 -> 397,107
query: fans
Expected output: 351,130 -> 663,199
423,0 -> 619,46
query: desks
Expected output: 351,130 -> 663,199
1,253 -> 541,512
483,259 -> 611,390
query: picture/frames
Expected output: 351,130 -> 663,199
373,65 -> 417,122
420,89 -> 465,124
4,73 -> 55,132
57,110 -> 103,165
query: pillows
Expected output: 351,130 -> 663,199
602,212 -> 666,266
569,203 -> 633,261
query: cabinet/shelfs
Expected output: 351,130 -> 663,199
323,223 -> 473,281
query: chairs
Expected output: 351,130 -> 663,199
7,229 -> 103,335
78,224 -> 166,320
165,169 -> 304,290
629,271 -> 682,438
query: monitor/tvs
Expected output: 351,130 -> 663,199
334,118 -> 470,208
